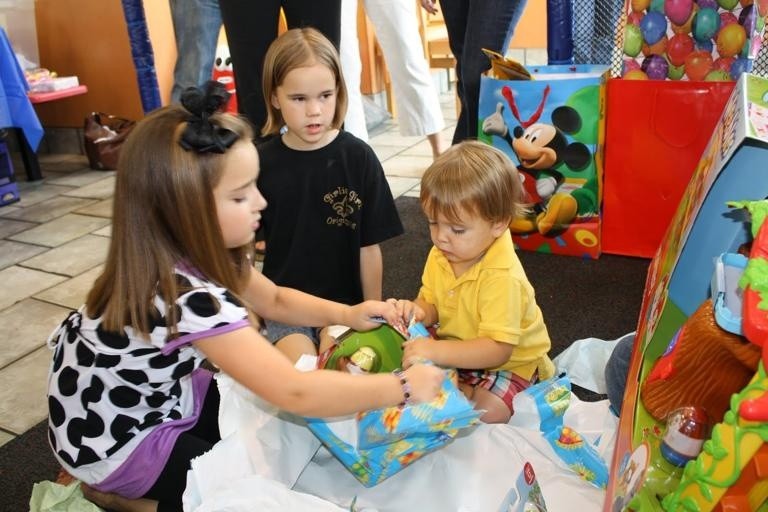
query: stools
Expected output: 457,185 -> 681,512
0,142 -> 20,206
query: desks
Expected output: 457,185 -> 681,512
8,84 -> 89,181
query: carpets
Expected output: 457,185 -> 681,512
0,196 -> 653,512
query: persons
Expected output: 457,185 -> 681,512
385,138 -> 559,426
249,27 -> 406,371
45,78 -> 447,512
336,0 -> 452,165
418,0 -> 531,147
168,1 -> 226,109
216,1 -> 344,139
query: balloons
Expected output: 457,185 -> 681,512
622,0 -> 766,83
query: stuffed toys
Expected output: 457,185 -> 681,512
482,99 -> 591,239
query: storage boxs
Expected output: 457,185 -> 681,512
600,76 -> 736,259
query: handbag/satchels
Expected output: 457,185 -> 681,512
83,109 -> 138,172
475,59 -> 613,262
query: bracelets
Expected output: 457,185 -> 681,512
392,369 -> 414,411
